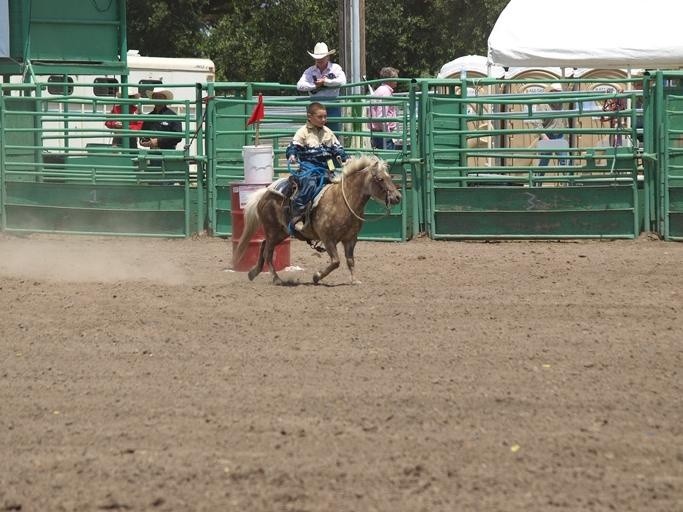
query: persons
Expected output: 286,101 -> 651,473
296,42 -> 346,140
105,87 -> 143,153
283,101 -> 354,235
533,82 -> 574,184
364,66 -> 401,150
137,86 -> 182,167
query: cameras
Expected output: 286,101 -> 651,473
328,73 -> 336,79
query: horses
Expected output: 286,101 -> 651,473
227,153 -> 404,288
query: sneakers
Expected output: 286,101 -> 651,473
293,218 -> 306,232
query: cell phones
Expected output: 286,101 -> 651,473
313,75 -> 317,82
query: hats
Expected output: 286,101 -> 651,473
306,41 -> 337,61
115,86 -> 140,100
545,83 -> 566,93
144,89 -> 175,103
